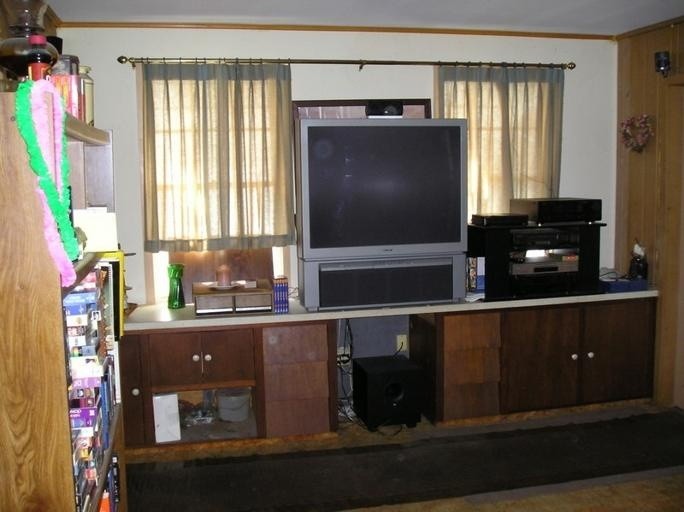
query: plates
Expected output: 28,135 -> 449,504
208,281 -> 240,290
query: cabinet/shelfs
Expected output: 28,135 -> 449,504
120,297 -> 654,449
2,92 -> 129,512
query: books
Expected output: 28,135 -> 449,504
65,251 -> 124,512
273,276 -> 290,314
465,256 -> 487,303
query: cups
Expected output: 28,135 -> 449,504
216,265 -> 232,287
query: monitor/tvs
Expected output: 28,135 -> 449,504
295,117 -> 468,262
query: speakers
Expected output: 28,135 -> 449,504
363,98 -> 405,117
353,355 -> 417,432
297,253 -> 472,312
655,50 -> 672,71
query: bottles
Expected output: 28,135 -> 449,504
26,34 -> 52,83
54,55 -> 94,128
168,263 -> 185,309
0,0 -> 59,92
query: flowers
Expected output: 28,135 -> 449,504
618,114 -> 656,156
